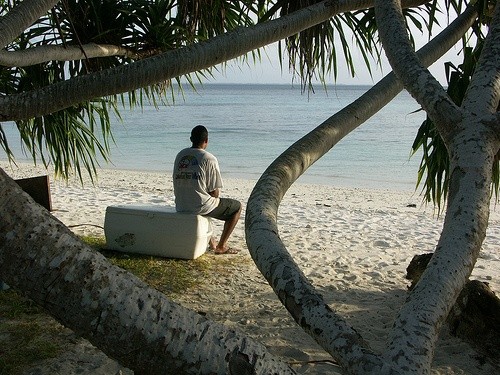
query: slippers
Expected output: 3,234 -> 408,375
208,240 -> 220,252
214,246 -> 238,256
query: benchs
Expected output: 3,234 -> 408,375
104,204 -> 211,259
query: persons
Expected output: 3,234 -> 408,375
172,124 -> 244,255
